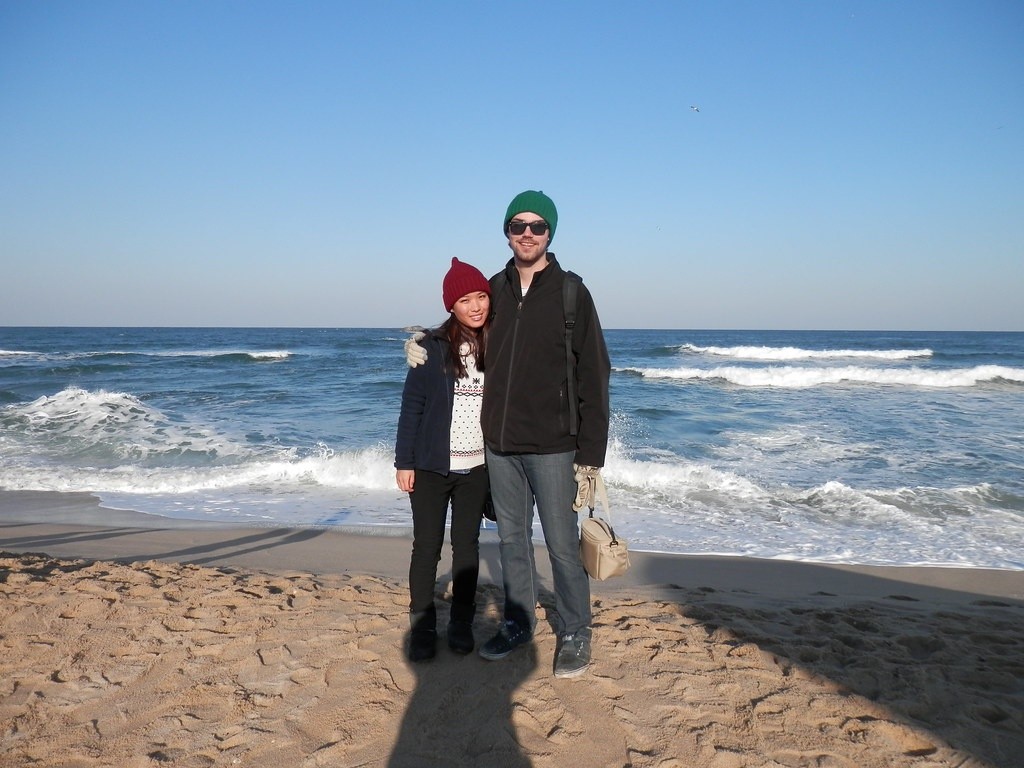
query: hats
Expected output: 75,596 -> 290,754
443,257 -> 492,313
504,190 -> 558,248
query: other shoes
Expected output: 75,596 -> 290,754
409,629 -> 437,663
447,615 -> 474,656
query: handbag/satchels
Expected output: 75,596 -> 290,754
572,466 -> 628,581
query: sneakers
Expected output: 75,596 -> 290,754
555,627 -> 592,678
479,620 -> 530,659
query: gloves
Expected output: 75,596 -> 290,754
403,332 -> 428,368
574,463 -> 604,480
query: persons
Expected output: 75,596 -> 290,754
392,257 -> 493,663
405,189 -> 611,679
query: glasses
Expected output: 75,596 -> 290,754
509,219 -> 548,235
